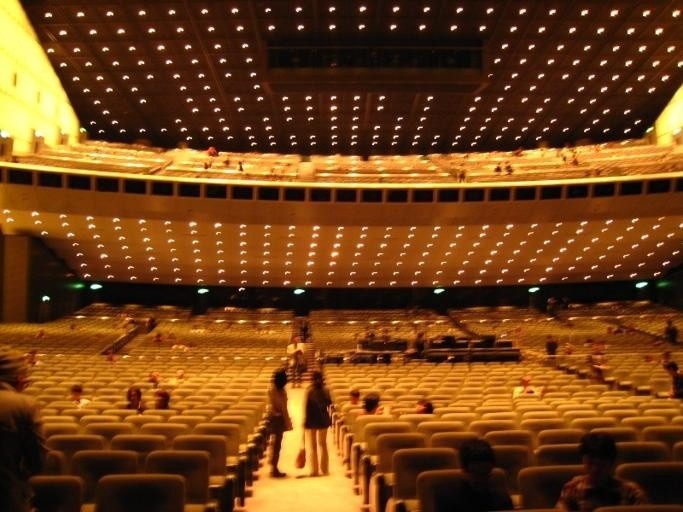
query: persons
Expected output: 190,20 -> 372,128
153,391 -> 167,408
303,370 -> 332,478
658,349 -> 675,368
263,369 -> 292,477
543,298 -> 640,385
201,144 -> 291,182
125,386 -> 150,415
412,331 -> 424,357
435,439 -> 514,510
514,376 -> 540,397
345,390 -> 362,405
286,336 -> 307,388
414,399 -> 433,415
364,326 -> 374,338
444,351 -> 455,362
664,318 -> 677,346
23,312 -> 198,388
448,130 -> 682,184
0,347 -> 64,511
379,327 -> 391,342
298,316 -> 309,341
361,393 -> 386,417
553,434 -> 646,512
664,360 -> 682,397
69,383 -> 87,403
